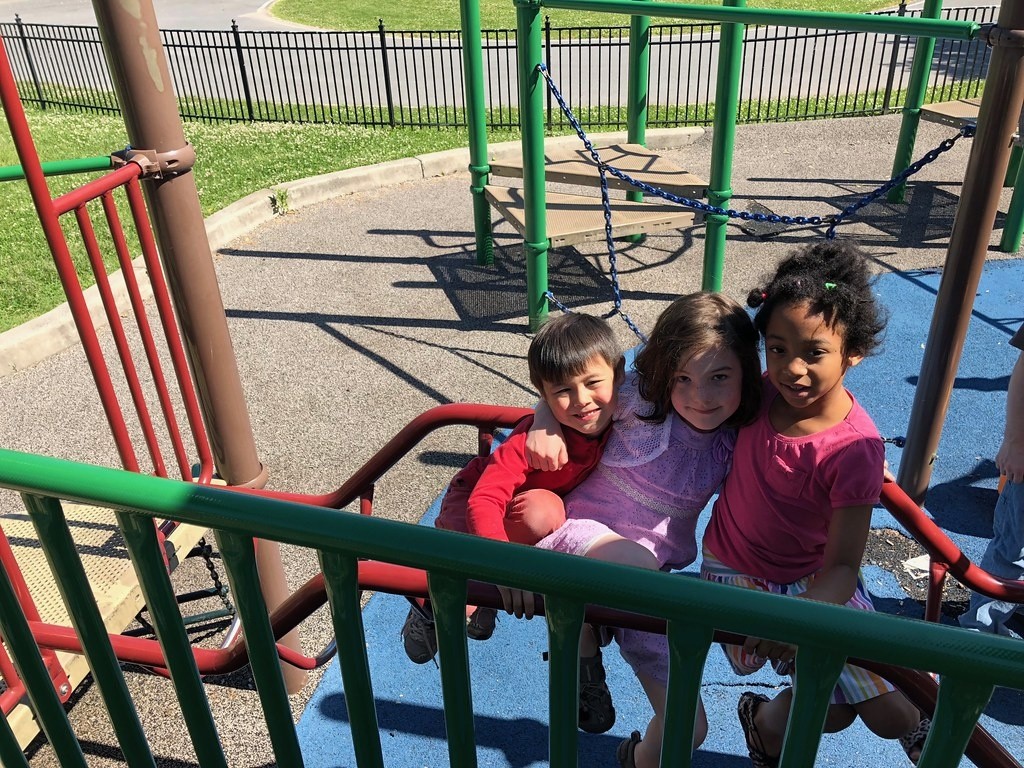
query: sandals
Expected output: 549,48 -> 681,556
900,720 -> 933,767
738,691 -> 779,768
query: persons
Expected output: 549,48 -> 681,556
403,313 -> 625,664
700,242 -> 934,768
525,292 -> 762,768
956,323 -> 1024,639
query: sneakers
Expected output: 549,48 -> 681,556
579,654 -> 616,732
615,731 -> 641,768
400,597 -> 438,664
467,607 -> 497,641
